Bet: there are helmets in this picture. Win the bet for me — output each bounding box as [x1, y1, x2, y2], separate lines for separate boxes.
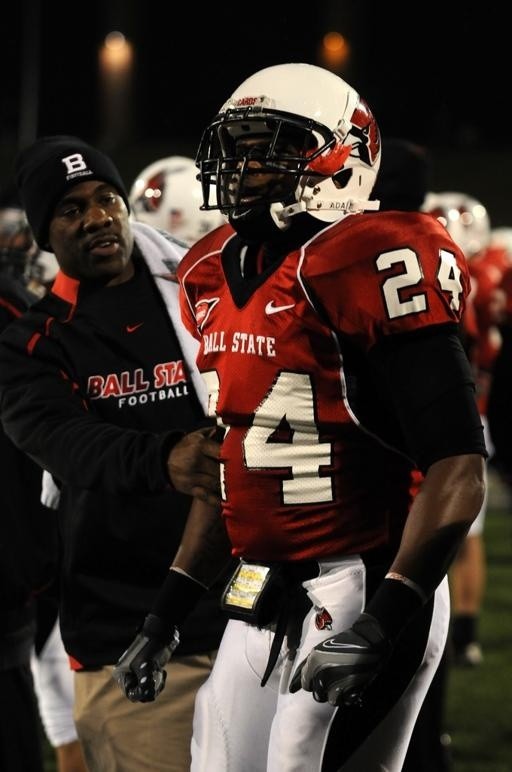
[418, 189, 492, 260]
[128, 156, 232, 252]
[195, 63, 385, 233]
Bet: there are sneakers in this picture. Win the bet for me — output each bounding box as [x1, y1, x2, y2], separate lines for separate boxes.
[453, 639, 485, 664]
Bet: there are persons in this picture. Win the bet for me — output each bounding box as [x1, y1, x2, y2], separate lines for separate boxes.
[117, 64, 486, 772]
[0, 139, 235, 771]
[370, 139, 510, 772]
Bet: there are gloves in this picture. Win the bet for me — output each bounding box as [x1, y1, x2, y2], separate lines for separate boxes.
[288, 611, 395, 712]
[113, 610, 180, 703]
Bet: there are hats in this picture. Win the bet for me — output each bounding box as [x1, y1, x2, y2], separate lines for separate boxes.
[23, 138, 130, 252]
